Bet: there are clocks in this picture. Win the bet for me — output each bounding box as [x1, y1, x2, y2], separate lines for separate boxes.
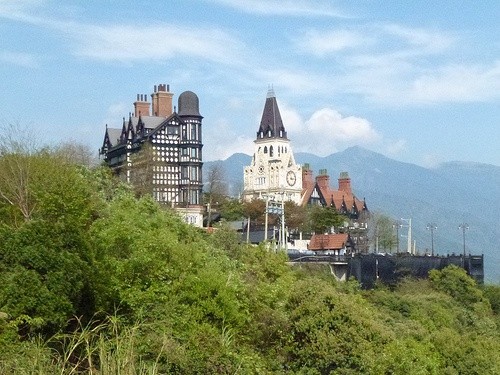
[287, 171, 296, 187]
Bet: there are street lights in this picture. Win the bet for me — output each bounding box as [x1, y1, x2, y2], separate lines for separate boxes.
[352, 218, 468, 255]
